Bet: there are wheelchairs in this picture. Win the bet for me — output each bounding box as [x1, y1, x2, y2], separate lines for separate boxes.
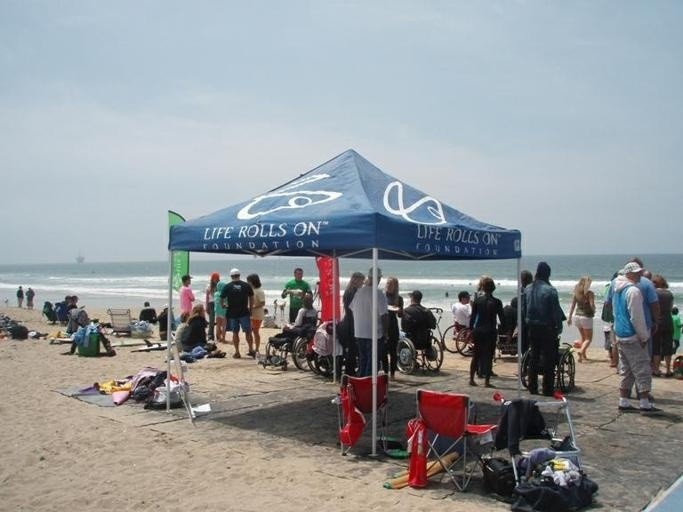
[256, 310, 360, 378]
[394, 306, 443, 372]
[442, 321, 477, 357]
[520, 340, 578, 394]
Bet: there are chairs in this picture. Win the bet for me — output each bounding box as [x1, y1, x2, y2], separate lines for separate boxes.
[406, 388, 499, 492]
[107, 308, 138, 337]
[42, 302, 99, 323]
[330, 371, 389, 457]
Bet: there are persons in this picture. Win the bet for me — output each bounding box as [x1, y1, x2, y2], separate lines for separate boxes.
[568, 275, 596, 362]
[175, 276, 207, 351]
[140, 301, 174, 340]
[336, 268, 403, 380]
[401, 290, 436, 349]
[62, 295, 71, 306]
[25, 288, 36, 310]
[206, 268, 265, 358]
[281, 268, 317, 339]
[69, 296, 79, 310]
[16, 286, 23, 308]
[601, 260, 682, 415]
[452, 261, 567, 396]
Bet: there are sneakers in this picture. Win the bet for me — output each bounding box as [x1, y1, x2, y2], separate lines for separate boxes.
[618, 405, 640, 413]
[639, 406, 663, 415]
[247, 352, 255, 356]
[666, 373, 676, 378]
[232, 352, 241, 358]
[469, 371, 498, 388]
[653, 371, 663, 377]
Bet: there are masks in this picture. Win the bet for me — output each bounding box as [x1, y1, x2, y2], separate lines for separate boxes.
[409, 291, 422, 298]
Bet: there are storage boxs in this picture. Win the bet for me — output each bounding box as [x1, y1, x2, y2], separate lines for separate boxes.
[77, 332, 101, 356]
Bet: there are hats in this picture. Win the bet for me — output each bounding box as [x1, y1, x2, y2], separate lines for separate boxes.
[625, 262, 644, 274]
[458, 291, 472, 297]
[230, 268, 240, 275]
